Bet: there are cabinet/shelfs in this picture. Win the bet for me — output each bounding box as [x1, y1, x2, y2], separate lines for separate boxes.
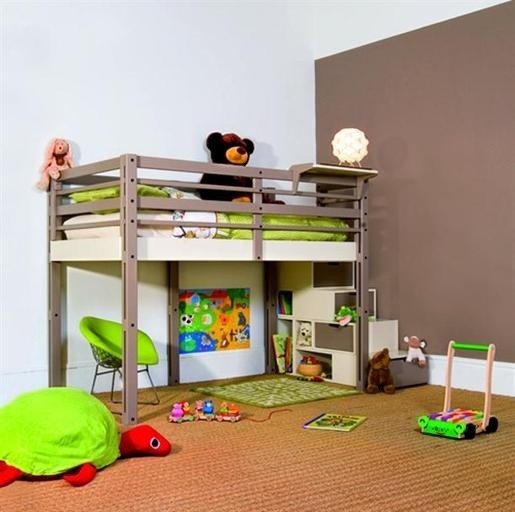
[272, 261, 430, 392]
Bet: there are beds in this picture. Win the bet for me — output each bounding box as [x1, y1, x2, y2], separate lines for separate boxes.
[47, 153, 370, 426]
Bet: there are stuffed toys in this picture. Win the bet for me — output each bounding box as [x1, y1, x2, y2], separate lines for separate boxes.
[198, 131, 287, 215]
[366, 347, 396, 395]
[403, 334, 427, 370]
[0, 386, 172, 488]
[35, 136, 77, 192]
[297, 323, 313, 347]
[334, 304, 375, 328]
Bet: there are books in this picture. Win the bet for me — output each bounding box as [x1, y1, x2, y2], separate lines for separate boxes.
[302, 412, 368, 433]
[271, 291, 294, 376]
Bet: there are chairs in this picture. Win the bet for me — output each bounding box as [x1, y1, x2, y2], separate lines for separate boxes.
[79, 315, 159, 416]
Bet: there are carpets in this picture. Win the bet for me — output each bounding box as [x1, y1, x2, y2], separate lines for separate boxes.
[188, 377, 360, 407]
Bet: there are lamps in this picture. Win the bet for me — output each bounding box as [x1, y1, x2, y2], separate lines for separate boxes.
[330, 128, 371, 167]
